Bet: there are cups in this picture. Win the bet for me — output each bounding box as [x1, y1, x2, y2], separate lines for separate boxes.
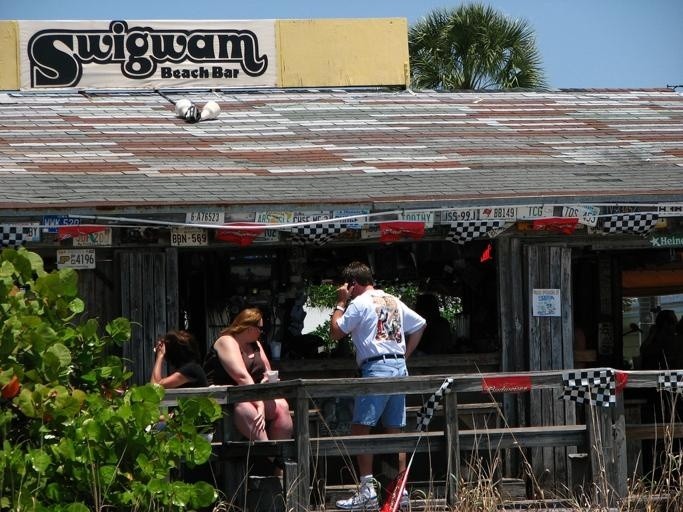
[268, 371, 278, 384]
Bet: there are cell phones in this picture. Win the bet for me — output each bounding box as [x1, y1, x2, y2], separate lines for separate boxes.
[346, 279, 355, 291]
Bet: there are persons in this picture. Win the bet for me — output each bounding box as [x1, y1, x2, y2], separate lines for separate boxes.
[641, 306, 683, 421]
[212, 306, 296, 475]
[329, 260, 428, 510]
[144, 327, 216, 482]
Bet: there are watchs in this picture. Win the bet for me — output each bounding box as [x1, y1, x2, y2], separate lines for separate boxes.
[333, 304, 346, 311]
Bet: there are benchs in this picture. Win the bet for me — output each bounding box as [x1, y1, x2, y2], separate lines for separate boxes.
[133, 368, 682, 508]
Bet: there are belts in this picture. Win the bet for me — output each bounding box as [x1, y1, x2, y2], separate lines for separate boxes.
[363, 355, 404, 364]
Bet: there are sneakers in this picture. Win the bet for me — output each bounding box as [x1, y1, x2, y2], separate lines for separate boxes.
[336, 492, 379, 509]
[399, 489, 409, 506]
